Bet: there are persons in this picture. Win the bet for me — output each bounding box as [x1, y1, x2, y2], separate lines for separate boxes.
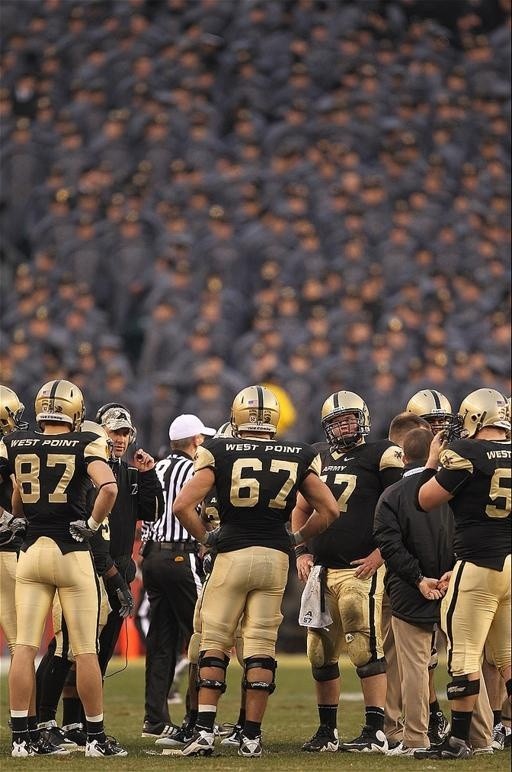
[382, 414, 432, 749]
[374, 430, 495, 759]
[38, 419, 136, 751]
[1, 2, 512, 389]
[172, 385, 341, 757]
[406, 391, 453, 747]
[0, 380, 128, 758]
[140, 412, 218, 738]
[290, 389, 403, 752]
[64, 405, 166, 748]
[416, 390, 512, 758]
[2, 386, 68, 755]
[155, 419, 281, 757]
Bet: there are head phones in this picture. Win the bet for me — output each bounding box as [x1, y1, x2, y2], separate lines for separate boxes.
[95, 403, 137, 442]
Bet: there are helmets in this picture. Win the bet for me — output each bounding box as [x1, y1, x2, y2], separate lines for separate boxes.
[231, 386, 280, 433]
[321, 391, 371, 448]
[449, 387, 510, 437]
[406, 389, 453, 439]
[212, 422, 234, 440]
[35, 380, 85, 431]
[0, 385, 29, 435]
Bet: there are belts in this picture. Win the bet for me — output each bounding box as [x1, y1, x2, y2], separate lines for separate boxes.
[160, 541, 193, 553]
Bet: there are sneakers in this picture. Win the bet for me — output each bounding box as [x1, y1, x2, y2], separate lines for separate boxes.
[212, 719, 234, 737]
[470, 746, 494, 756]
[37, 720, 78, 750]
[85, 736, 128, 758]
[154, 723, 198, 746]
[340, 725, 389, 753]
[220, 725, 243, 747]
[430, 709, 451, 745]
[382, 742, 429, 757]
[181, 730, 215, 756]
[141, 719, 181, 739]
[414, 737, 473, 761]
[301, 725, 340, 754]
[237, 735, 263, 758]
[9, 730, 52, 759]
[28, 728, 70, 757]
[490, 723, 511, 751]
[60, 725, 88, 752]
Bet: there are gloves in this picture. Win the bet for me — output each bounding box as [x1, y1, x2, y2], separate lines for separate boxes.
[1, 510, 28, 536]
[110, 572, 136, 620]
[201, 529, 221, 549]
[67, 520, 94, 543]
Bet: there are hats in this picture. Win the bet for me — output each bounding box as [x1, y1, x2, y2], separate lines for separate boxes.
[168, 414, 216, 440]
[96, 403, 134, 432]
